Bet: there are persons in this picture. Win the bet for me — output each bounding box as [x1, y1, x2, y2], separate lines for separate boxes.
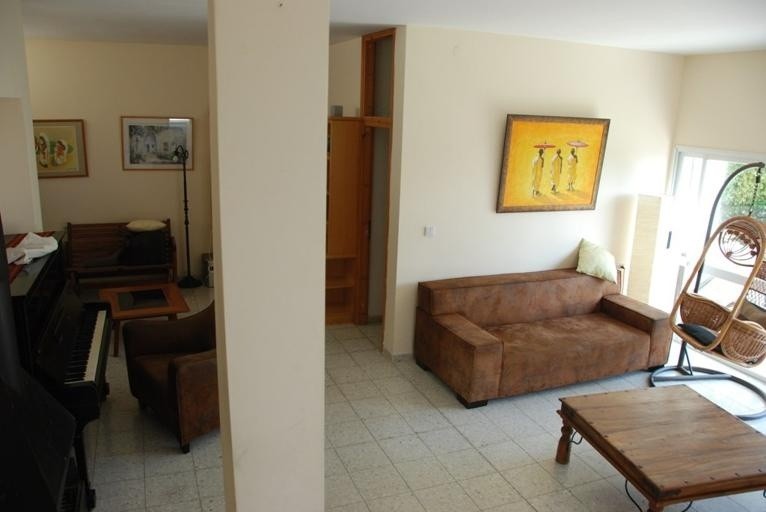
[566, 148, 578, 191]
[531, 148, 544, 196]
[550, 148, 563, 192]
[56, 140, 65, 160]
[38, 136, 48, 168]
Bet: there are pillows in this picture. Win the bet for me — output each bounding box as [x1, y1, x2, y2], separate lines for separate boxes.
[576, 237, 618, 283]
[677, 323, 721, 352]
[126, 220, 167, 232]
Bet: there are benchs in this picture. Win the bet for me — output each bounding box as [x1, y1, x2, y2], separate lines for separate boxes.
[67, 219, 178, 298]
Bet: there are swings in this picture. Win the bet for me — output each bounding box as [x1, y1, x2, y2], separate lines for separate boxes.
[669, 165, 765, 369]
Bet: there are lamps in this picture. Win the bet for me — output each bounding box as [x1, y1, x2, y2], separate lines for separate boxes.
[172, 144, 203, 289]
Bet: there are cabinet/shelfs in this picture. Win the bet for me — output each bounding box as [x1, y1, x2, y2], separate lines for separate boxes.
[325, 116, 359, 326]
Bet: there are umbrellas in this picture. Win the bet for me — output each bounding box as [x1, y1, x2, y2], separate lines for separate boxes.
[533, 143, 555, 148]
[566, 140, 589, 147]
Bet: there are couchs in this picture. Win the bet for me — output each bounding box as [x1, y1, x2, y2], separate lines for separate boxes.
[413, 267, 677, 410]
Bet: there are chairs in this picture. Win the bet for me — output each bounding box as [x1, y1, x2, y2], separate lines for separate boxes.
[122, 300, 220, 454]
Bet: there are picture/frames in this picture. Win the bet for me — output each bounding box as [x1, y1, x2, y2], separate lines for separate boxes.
[495, 114, 611, 214]
[32, 118, 89, 179]
[120, 116, 194, 171]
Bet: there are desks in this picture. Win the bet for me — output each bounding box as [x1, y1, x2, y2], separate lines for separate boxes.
[98, 282, 190, 357]
